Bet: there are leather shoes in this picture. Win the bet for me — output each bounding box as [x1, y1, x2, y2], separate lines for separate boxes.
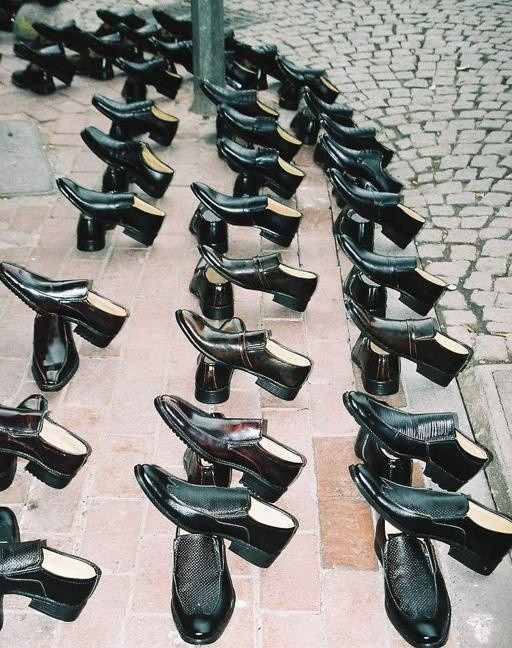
[0, 234, 512, 648]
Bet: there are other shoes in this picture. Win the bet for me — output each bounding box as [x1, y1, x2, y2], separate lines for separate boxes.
[9, 7, 425, 253]
